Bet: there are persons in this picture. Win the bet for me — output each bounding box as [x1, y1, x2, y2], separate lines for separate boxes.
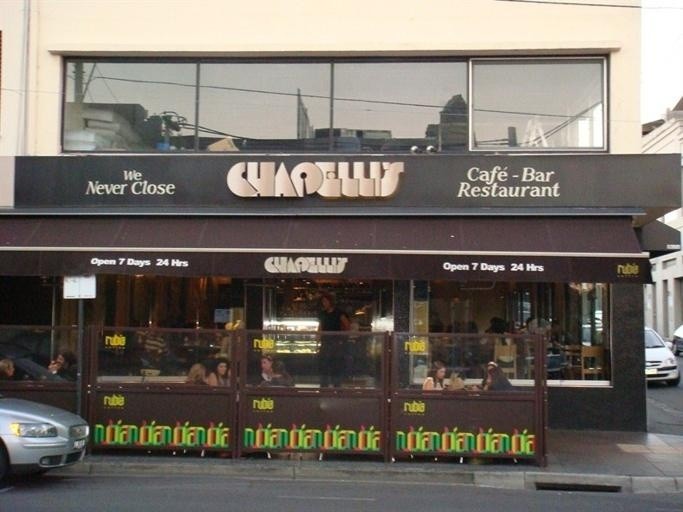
[0, 357, 17, 380]
[254, 352, 294, 386]
[184, 362, 209, 385]
[420, 359, 448, 391]
[43, 350, 78, 382]
[429, 311, 564, 377]
[469, 367, 512, 392]
[311, 293, 352, 388]
[204, 356, 232, 387]
[260, 360, 292, 386]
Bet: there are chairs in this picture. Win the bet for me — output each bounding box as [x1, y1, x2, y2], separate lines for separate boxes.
[494, 344, 607, 380]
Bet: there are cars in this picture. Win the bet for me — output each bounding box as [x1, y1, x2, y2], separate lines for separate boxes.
[0, 396, 90, 486]
[517, 301, 531, 321]
[583, 310, 605, 344]
[645, 325, 683, 387]
